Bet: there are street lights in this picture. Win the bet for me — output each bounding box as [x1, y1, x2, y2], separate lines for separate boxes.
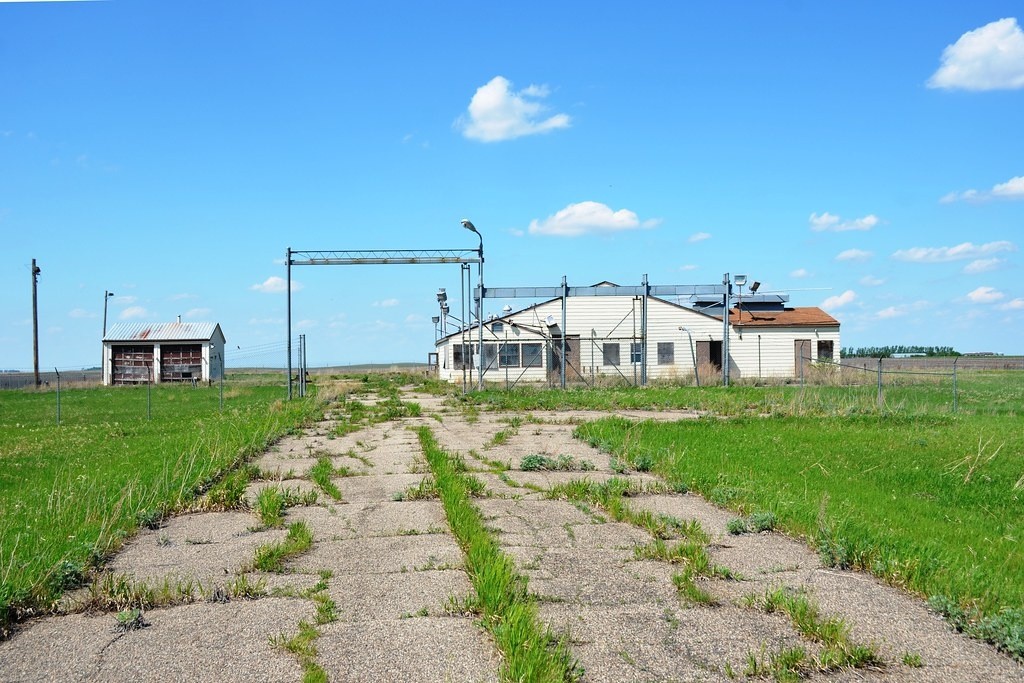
[101, 290, 114, 381]
[432, 316, 440, 342]
[460, 219, 483, 388]
